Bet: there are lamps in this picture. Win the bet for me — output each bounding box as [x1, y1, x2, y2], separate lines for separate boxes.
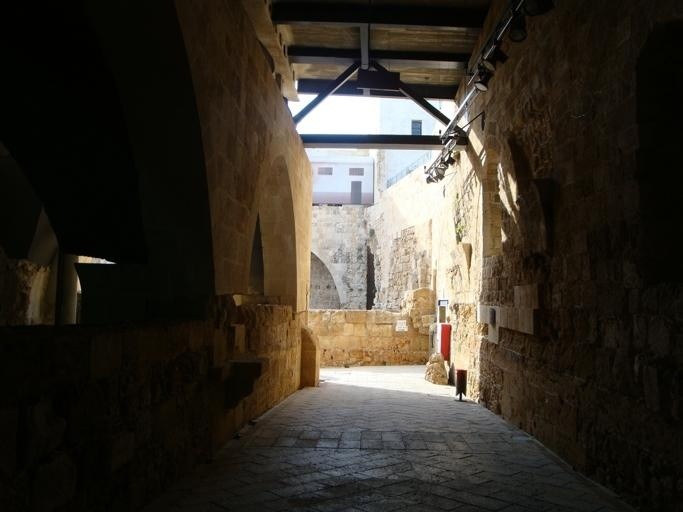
[424, 0, 554, 185]
